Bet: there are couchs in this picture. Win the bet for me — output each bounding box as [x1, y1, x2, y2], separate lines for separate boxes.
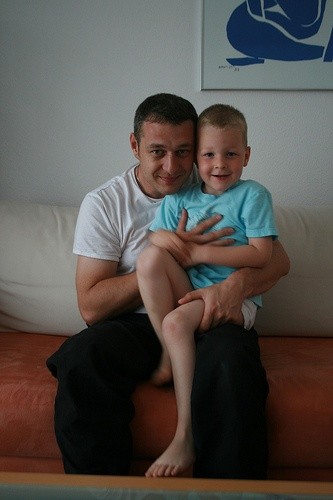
[0, 202, 333, 480]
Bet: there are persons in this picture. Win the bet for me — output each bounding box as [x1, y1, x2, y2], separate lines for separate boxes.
[135, 105, 278, 478]
[46, 92, 289, 480]
